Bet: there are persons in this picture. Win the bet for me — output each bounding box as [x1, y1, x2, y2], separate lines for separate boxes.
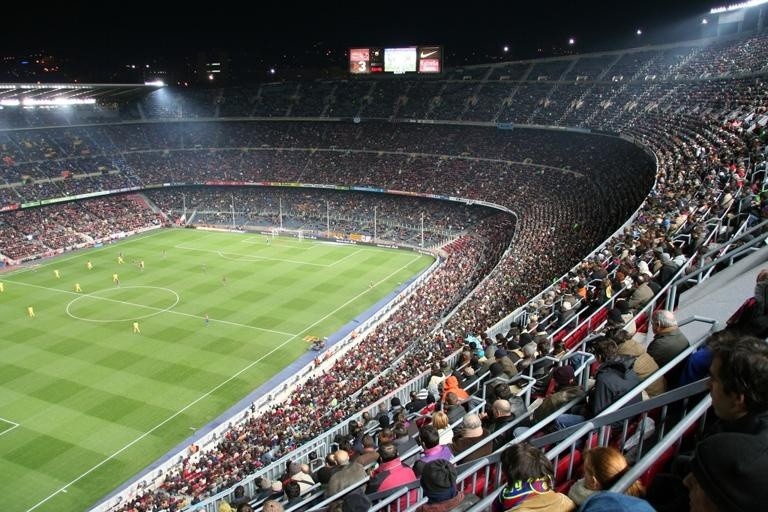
[27, 306, 35, 317]
[75, 283, 82, 293]
[108, 275, 768, 510]
[0, 283, 4, 293]
[0, 34, 768, 273]
[54, 269, 61, 279]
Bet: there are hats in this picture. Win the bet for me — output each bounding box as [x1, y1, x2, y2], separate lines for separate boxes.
[580, 493, 656, 512]
[554, 366, 576, 386]
[420, 458, 459, 498]
[289, 463, 302, 474]
[689, 432, 767, 511]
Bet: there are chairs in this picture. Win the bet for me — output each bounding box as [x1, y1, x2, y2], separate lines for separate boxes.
[218, 159, 768, 512]
